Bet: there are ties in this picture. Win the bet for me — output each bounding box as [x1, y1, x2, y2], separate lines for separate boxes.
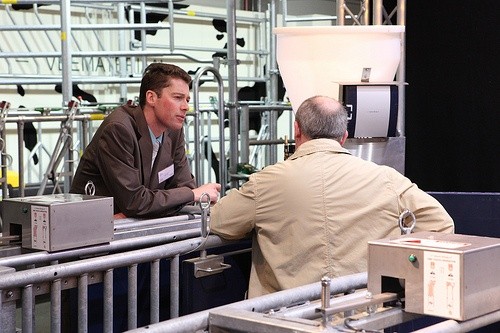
[152, 141, 159, 173]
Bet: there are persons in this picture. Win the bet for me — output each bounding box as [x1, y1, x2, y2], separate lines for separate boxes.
[208, 95, 456, 333]
[68, 62, 222, 220]
